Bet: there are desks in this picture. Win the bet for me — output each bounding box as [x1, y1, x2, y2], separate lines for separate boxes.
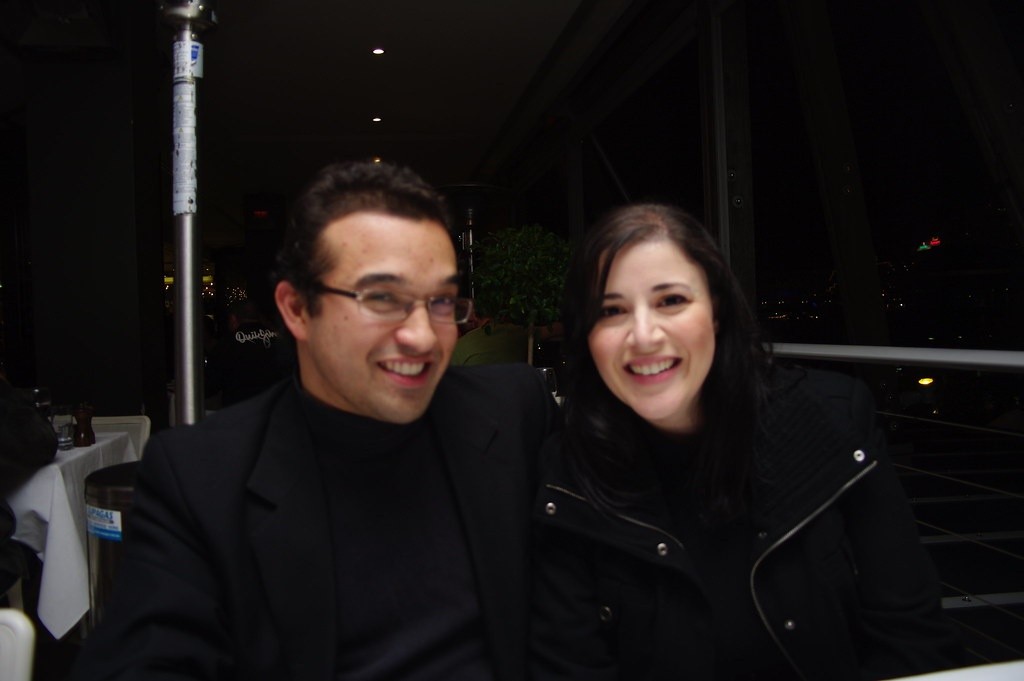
[4, 430, 137, 643]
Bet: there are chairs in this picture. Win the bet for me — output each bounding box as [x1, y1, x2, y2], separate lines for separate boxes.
[48, 416, 151, 461]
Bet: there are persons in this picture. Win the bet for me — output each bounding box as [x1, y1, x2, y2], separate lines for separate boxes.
[0, 368, 81, 681]
[450, 274, 543, 367]
[74, 157, 564, 681]
[520, 203, 969, 681]
[206, 297, 286, 398]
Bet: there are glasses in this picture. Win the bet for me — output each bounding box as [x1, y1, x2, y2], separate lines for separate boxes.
[315, 284, 473, 323]
